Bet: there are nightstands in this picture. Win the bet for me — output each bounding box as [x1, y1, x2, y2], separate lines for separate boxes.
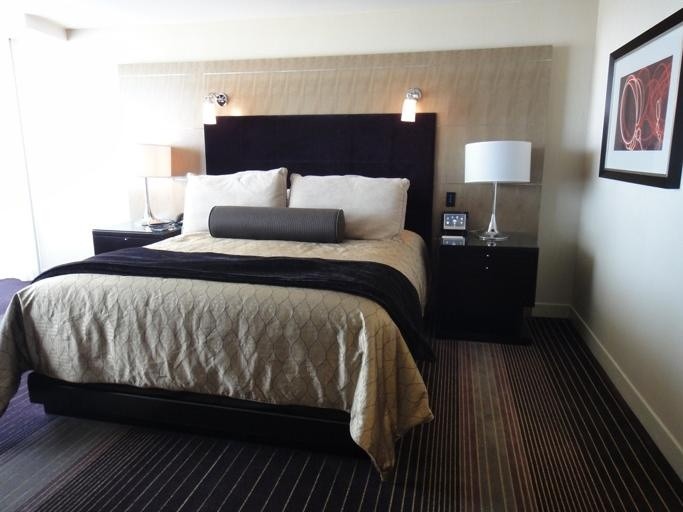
[91, 227, 183, 255]
[439, 235, 540, 344]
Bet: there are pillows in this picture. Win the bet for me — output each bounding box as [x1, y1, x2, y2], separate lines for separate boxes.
[183, 169, 409, 245]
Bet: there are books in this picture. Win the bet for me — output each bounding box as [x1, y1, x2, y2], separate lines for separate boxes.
[148, 220, 173, 232]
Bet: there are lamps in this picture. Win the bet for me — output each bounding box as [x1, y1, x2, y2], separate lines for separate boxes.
[201, 90, 423, 123]
[115, 143, 172, 232]
[464, 142, 532, 241]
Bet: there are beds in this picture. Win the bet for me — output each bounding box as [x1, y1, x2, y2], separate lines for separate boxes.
[15, 112, 437, 449]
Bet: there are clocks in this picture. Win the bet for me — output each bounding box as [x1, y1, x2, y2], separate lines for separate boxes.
[441, 210, 467, 238]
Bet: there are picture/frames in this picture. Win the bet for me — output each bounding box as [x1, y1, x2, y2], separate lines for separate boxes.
[598, 7, 682, 190]
